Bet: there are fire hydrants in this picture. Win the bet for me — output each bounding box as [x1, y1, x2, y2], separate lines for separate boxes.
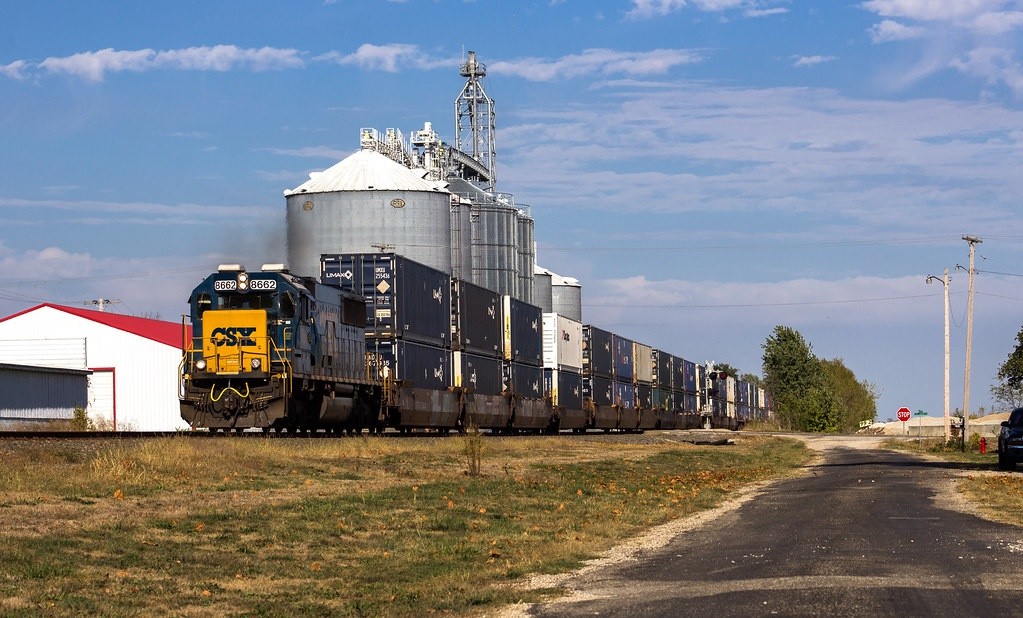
[979, 437, 987, 453]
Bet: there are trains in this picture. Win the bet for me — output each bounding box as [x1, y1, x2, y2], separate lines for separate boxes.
[178, 252, 773, 436]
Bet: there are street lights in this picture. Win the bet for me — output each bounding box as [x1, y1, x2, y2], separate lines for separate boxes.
[955, 264, 974, 452]
[926, 274, 950, 447]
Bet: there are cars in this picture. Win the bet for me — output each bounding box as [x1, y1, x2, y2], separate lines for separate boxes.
[998, 407, 1023, 471]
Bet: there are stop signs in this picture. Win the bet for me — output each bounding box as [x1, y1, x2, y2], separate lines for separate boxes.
[896, 407, 911, 422]
